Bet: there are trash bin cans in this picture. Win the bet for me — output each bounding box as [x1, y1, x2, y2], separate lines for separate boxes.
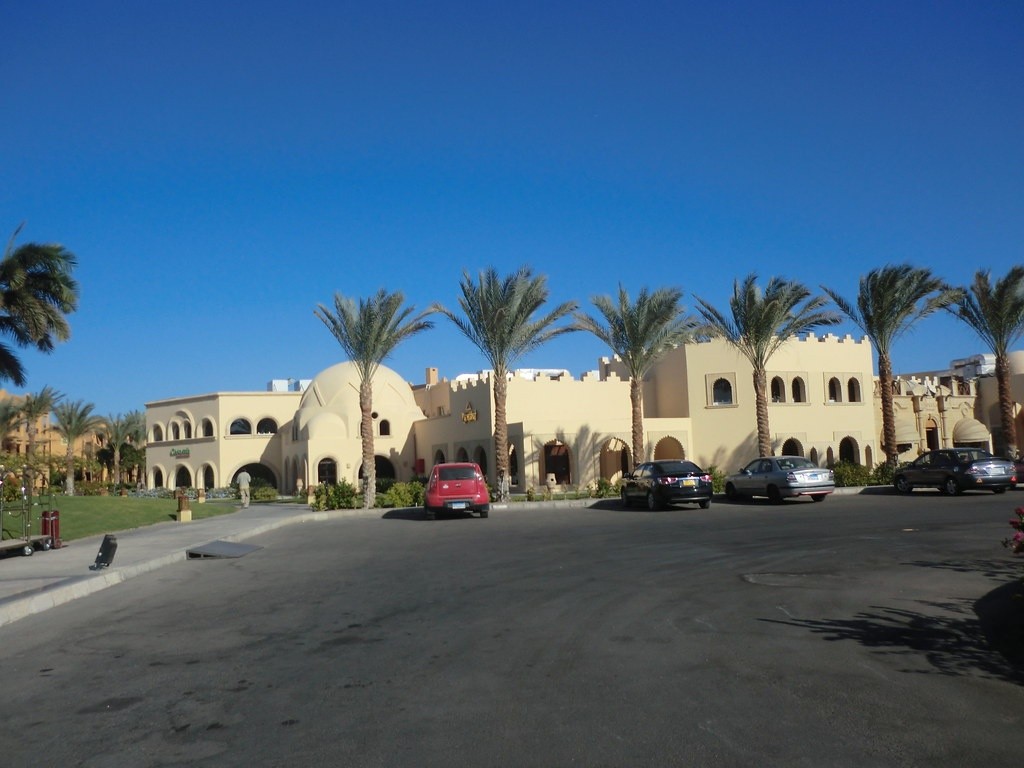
[41, 511, 59, 549]
[1014, 460, 1024, 483]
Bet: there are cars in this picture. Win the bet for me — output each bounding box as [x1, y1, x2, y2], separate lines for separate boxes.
[893, 447, 1017, 496]
[722, 456, 835, 505]
[620, 459, 714, 511]
[423, 460, 491, 520]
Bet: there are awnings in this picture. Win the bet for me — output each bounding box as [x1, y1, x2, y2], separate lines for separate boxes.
[953, 418, 990, 443]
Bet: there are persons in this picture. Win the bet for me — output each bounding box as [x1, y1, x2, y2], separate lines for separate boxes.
[236, 467, 252, 508]
